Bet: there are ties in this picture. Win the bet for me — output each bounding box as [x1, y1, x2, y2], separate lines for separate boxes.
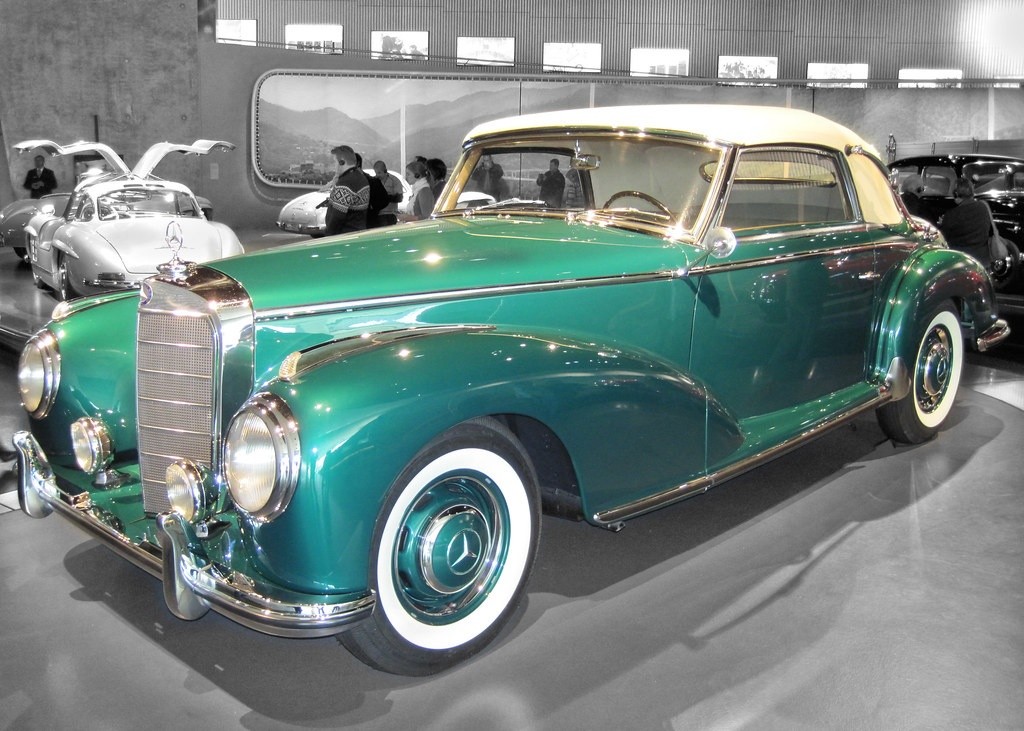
[38, 168, 41, 177]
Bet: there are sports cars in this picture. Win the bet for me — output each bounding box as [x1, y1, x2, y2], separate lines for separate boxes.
[1, 136, 248, 299]
[275, 168, 410, 241]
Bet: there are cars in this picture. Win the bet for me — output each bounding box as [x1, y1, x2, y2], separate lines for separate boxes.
[882, 152, 1024, 322]
[0, 104, 1011, 682]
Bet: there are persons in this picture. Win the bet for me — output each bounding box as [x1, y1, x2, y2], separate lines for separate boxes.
[373, 161, 403, 226]
[23, 155, 57, 199]
[356, 153, 389, 229]
[380, 34, 425, 60]
[724, 60, 765, 79]
[899, 174, 925, 217]
[399, 162, 435, 222]
[936, 177, 992, 274]
[425, 158, 447, 201]
[415, 156, 427, 164]
[472, 154, 503, 197]
[536, 158, 565, 207]
[325, 145, 370, 235]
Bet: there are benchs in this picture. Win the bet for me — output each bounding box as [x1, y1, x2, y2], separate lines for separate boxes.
[680, 203, 847, 232]
